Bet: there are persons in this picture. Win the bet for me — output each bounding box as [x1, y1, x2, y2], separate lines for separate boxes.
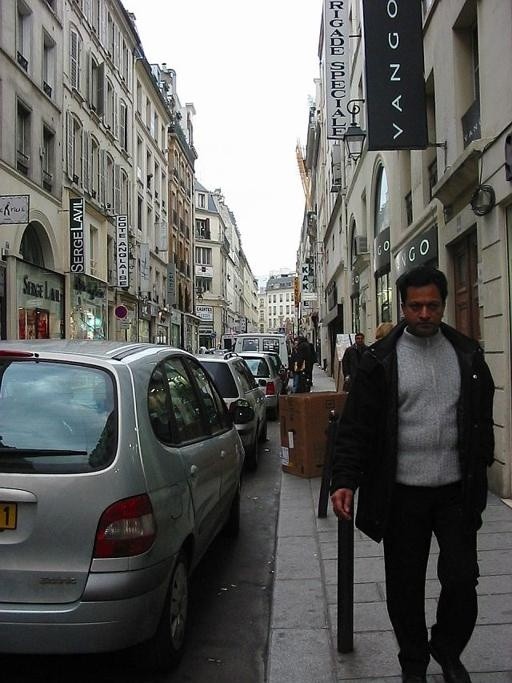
[291, 336, 316, 392]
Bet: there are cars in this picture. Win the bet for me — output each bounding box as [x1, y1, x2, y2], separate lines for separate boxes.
[190, 349, 290, 448]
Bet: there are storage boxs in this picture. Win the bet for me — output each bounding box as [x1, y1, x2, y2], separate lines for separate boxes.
[331, 331, 358, 392]
[277, 390, 350, 479]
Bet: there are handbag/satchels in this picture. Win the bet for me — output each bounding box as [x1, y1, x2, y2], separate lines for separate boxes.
[343, 377, 352, 392]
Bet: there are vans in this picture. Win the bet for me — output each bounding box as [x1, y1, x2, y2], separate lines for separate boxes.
[233, 332, 292, 375]
[0, 337, 246, 671]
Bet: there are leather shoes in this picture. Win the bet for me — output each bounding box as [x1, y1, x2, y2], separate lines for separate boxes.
[399, 640, 471, 683]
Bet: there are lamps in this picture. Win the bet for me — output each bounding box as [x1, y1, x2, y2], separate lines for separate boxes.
[341, 98, 367, 165]
